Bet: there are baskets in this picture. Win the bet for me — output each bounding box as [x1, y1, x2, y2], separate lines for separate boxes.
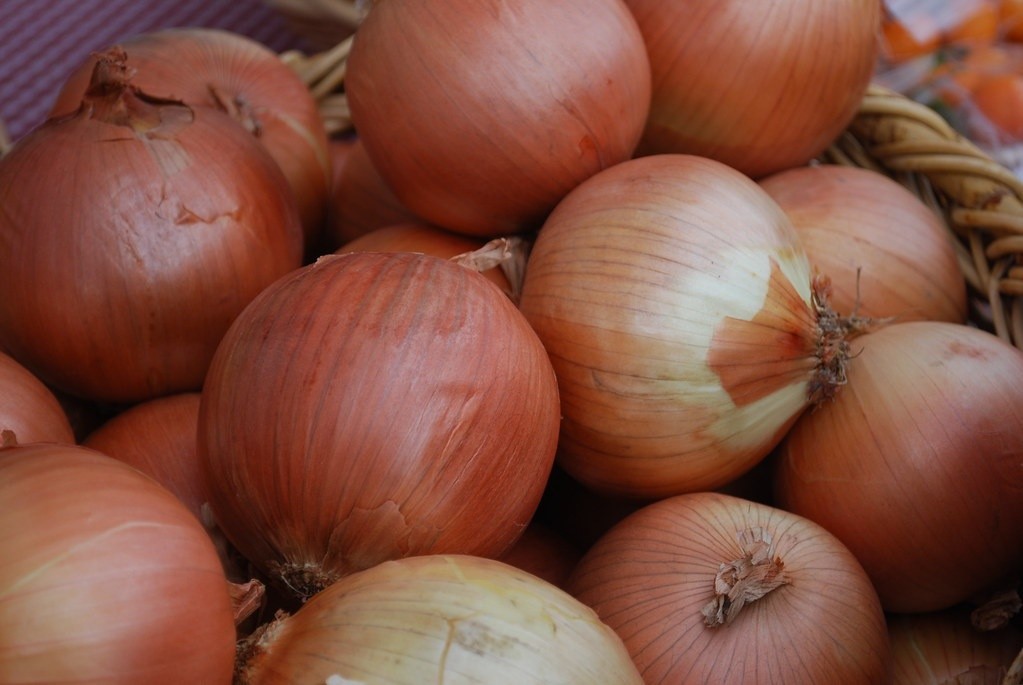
[279, 39, 1022, 685]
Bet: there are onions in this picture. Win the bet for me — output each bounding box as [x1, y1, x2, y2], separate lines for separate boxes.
[0, 0, 1023, 685]
